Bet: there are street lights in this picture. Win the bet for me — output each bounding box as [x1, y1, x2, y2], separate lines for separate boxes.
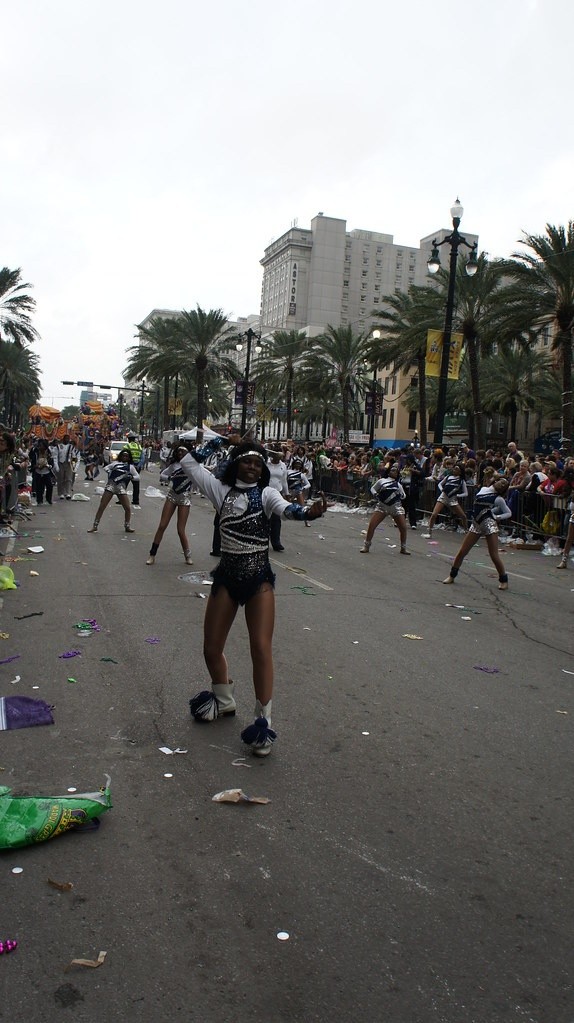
[204, 383, 213, 420]
[426, 196, 479, 444]
[368, 329, 381, 448]
[115, 393, 126, 418]
[235, 327, 263, 441]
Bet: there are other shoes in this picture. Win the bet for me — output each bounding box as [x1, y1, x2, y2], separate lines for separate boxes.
[394, 523, 397, 527]
[273, 544, 284, 551]
[87, 527, 97, 532]
[411, 525, 417, 530]
[145, 554, 156, 564]
[184, 551, 193, 565]
[66, 494, 71, 500]
[131, 501, 139, 505]
[37, 501, 43, 506]
[116, 501, 122, 504]
[124, 526, 136, 532]
[46, 499, 53, 505]
[60, 494, 64, 499]
[306, 523, 312, 527]
[210, 549, 222, 557]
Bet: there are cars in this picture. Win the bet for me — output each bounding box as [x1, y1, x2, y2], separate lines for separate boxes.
[101, 440, 130, 467]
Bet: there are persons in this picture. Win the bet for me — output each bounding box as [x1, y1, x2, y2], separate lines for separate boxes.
[84, 449, 99, 481]
[116, 436, 145, 505]
[556, 494, 574, 568]
[146, 444, 195, 565]
[0, 428, 81, 527]
[87, 450, 141, 533]
[35, 415, 64, 425]
[443, 476, 512, 590]
[139, 439, 236, 498]
[262, 438, 574, 551]
[360, 466, 411, 555]
[80, 403, 90, 415]
[178, 441, 336, 755]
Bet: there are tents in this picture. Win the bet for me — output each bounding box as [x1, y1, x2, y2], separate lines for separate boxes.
[178, 422, 221, 440]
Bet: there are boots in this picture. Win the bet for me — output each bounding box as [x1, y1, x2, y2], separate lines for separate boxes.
[188, 680, 236, 722]
[360, 541, 371, 553]
[427, 527, 433, 539]
[400, 544, 411, 555]
[556, 554, 569, 568]
[240, 699, 277, 756]
[442, 567, 459, 583]
[498, 573, 508, 589]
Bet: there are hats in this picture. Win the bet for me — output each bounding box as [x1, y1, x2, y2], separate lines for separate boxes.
[404, 454, 415, 460]
[544, 460, 557, 468]
[126, 430, 138, 437]
[459, 443, 468, 449]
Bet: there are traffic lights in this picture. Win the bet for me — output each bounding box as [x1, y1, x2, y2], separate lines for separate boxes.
[293, 408, 302, 414]
[271, 408, 280, 412]
